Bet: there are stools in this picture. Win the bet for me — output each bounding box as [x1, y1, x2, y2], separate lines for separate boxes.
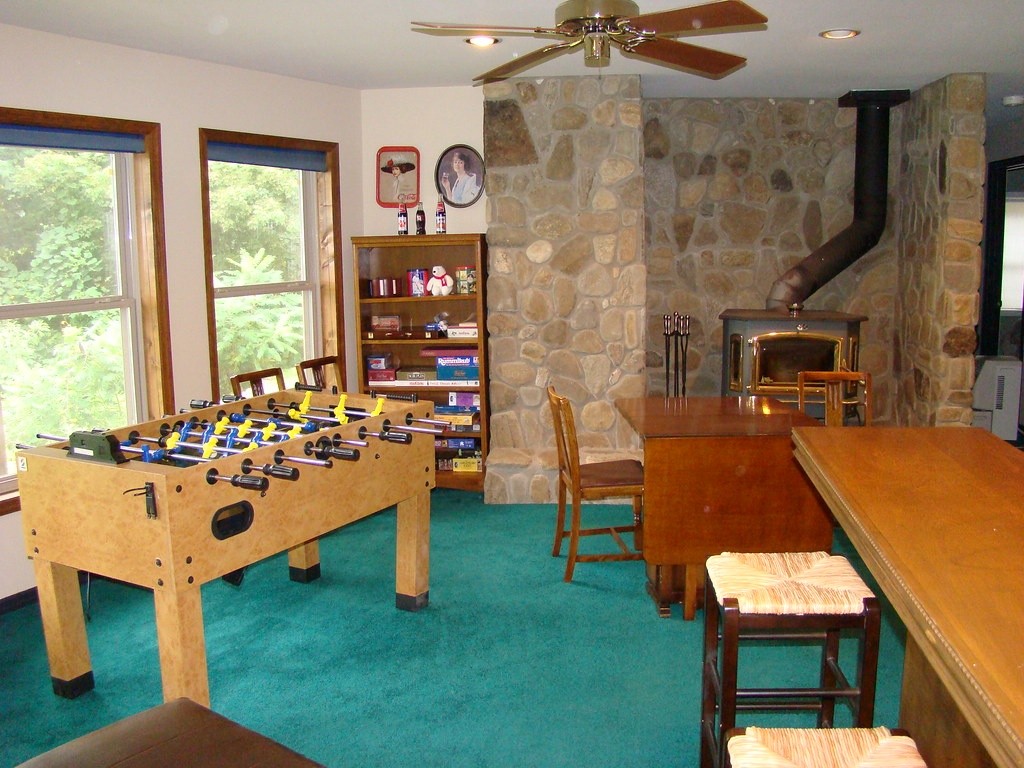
[701, 550, 879, 768]
[720, 726, 931, 768]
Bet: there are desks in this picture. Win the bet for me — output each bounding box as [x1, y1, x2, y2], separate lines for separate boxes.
[12, 696, 330, 768]
[612, 396, 834, 622]
[790, 425, 1024, 768]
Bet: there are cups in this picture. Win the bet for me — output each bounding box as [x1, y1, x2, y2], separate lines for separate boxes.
[443, 172, 449, 181]
[404, 268, 430, 297]
[454, 265, 476, 294]
[370, 277, 396, 297]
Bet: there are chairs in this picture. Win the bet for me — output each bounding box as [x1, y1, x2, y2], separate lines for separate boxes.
[798, 371, 872, 429]
[229, 367, 286, 401]
[546, 384, 643, 581]
[296, 356, 344, 392]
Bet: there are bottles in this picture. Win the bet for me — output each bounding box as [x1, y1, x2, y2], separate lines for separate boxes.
[415, 201, 426, 235]
[436, 194, 446, 234]
[397, 194, 409, 235]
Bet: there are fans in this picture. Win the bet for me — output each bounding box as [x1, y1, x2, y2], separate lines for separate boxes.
[410, 0, 768, 79]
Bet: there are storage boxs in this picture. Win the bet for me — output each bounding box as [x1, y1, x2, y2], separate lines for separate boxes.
[366, 346, 480, 380]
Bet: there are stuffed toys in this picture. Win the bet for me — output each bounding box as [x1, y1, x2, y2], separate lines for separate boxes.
[427, 265, 453, 296]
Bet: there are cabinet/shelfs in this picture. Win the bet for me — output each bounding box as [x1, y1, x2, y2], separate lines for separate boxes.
[350, 233, 486, 492]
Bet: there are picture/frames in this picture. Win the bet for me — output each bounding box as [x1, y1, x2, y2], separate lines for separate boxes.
[376, 145, 421, 208]
[434, 144, 486, 208]
